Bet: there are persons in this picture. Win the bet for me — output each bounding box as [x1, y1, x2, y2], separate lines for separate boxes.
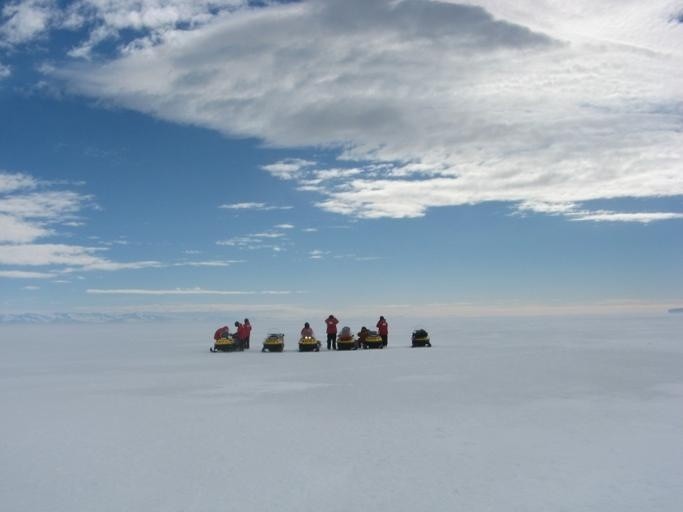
[300, 321, 314, 337]
[243, 317, 252, 350]
[209, 325, 230, 352]
[232, 320, 247, 352]
[337, 325, 355, 342]
[324, 314, 339, 350]
[357, 326, 369, 350]
[375, 315, 389, 349]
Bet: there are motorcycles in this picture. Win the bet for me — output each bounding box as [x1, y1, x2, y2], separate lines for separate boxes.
[411, 329, 431, 347]
[298, 336, 319, 352]
[362, 331, 382, 349]
[261, 334, 284, 352]
[336, 334, 356, 351]
[209, 334, 240, 352]
